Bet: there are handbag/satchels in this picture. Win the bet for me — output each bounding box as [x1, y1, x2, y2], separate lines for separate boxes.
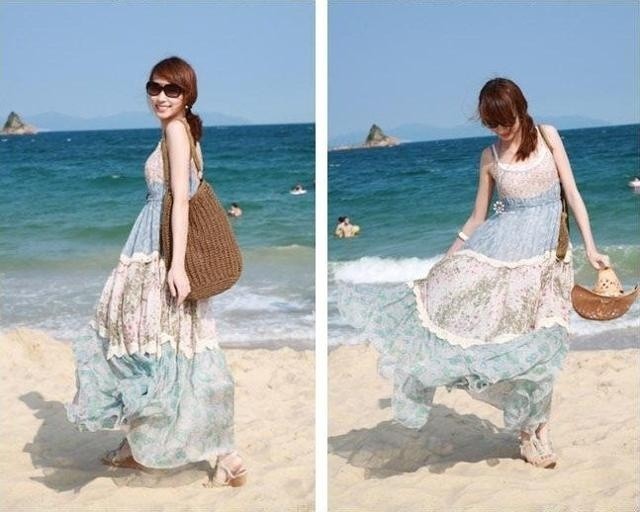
[161, 178, 243, 303]
[556, 212, 569, 263]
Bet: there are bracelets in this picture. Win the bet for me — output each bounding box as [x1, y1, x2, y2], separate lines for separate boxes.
[458, 232, 469, 241]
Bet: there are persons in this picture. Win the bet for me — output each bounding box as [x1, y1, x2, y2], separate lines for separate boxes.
[226, 201, 242, 218]
[628, 176, 639, 193]
[336, 77, 610, 470]
[64, 56, 249, 488]
[292, 185, 303, 196]
[336, 214, 354, 238]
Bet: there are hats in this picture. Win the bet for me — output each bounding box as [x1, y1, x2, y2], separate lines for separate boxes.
[570, 268, 640, 321]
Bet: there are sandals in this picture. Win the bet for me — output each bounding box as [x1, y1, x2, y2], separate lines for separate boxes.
[101, 438, 144, 470]
[203, 451, 247, 488]
[518, 429, 556, 470]
